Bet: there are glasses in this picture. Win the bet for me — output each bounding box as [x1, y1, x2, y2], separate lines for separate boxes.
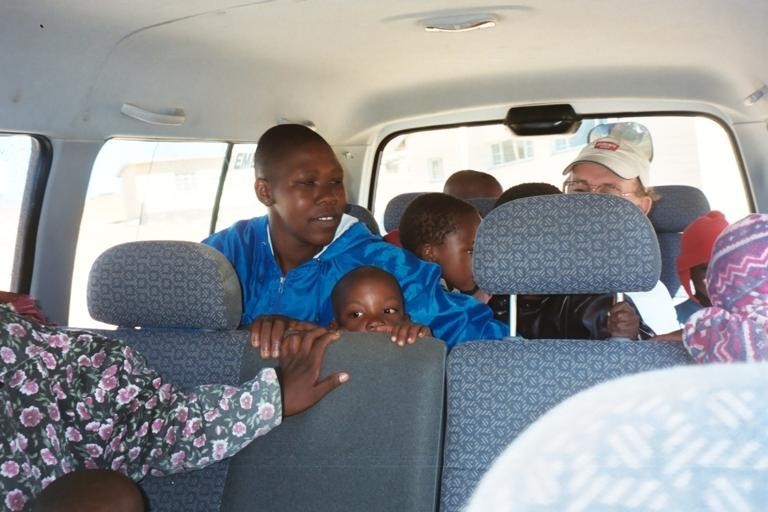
[562, 179, 633, 201]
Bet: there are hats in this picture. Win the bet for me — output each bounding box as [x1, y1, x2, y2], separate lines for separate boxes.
[466, 361, 768, 511]
[675, 210, 731, 305]
[562, 135, 652, 194]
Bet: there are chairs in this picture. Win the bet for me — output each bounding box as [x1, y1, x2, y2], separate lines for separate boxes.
[384, 191, 499, 236]
[79, 241, 448, 512]
[648, 186, 712, 322]
[438, 193, 698, 512]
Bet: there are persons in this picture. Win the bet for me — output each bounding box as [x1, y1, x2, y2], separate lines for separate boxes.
[0, 286, 348, 512]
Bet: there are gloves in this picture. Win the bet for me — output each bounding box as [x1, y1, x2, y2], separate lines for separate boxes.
[681, 211, 767, 362]
